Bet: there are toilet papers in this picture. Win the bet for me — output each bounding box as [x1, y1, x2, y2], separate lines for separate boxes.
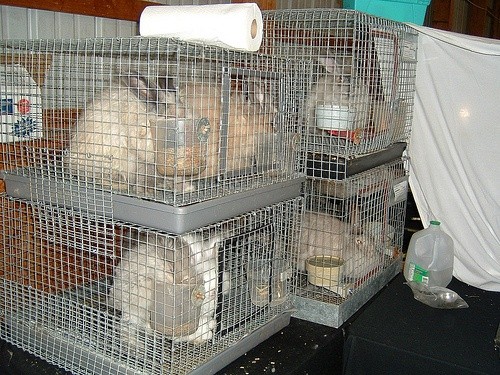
[139, 3, 264, 52]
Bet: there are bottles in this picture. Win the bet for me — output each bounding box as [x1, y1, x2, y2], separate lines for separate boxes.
[402, 221, 455, 291]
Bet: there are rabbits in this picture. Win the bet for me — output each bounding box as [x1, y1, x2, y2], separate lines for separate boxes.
[178, 80, 280, 180]
[66, 69, 195, 198]
[303, 49, 372, 134]
[108, 229, 235, 360]
[296, 205, 382, 278]
